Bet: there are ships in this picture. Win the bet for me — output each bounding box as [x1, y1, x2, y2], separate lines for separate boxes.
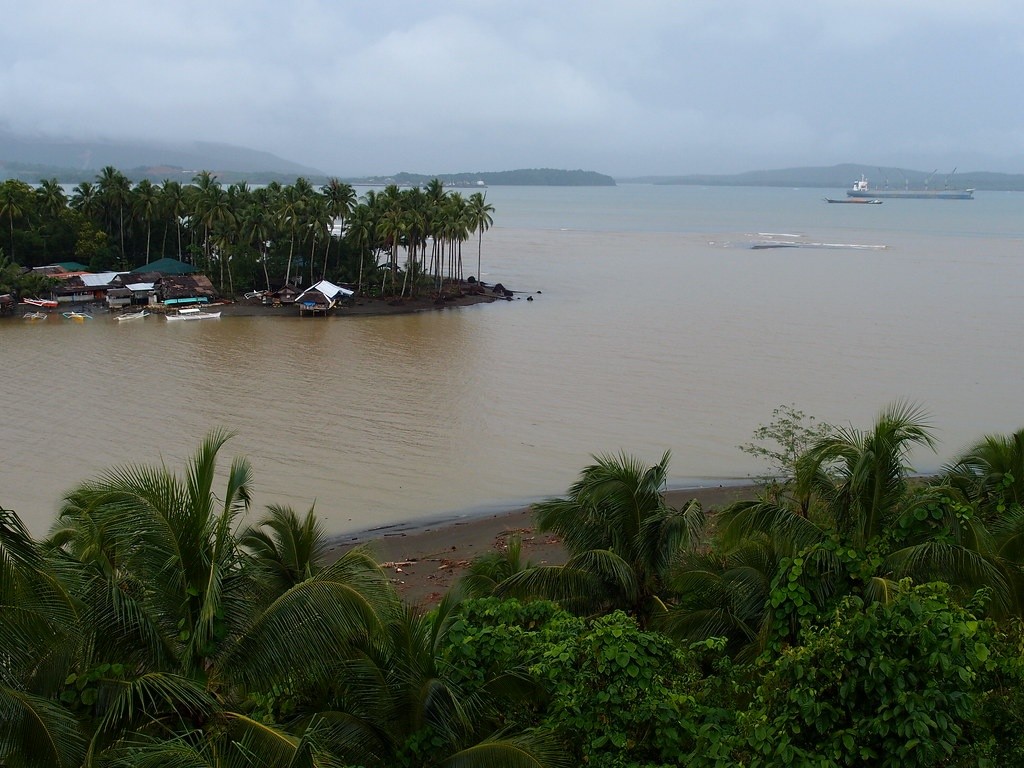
[847, 168, 975, 199]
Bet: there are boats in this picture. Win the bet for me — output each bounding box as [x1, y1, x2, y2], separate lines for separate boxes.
[826, 197, 882, 203]
[22, 312, 46, 320]
[25, 296, 58, 307]
[757, 232, 887, 248]
[113, 310, 149, 321]
[165, 308, 221, 321]
[64, 310, 93, 321]
